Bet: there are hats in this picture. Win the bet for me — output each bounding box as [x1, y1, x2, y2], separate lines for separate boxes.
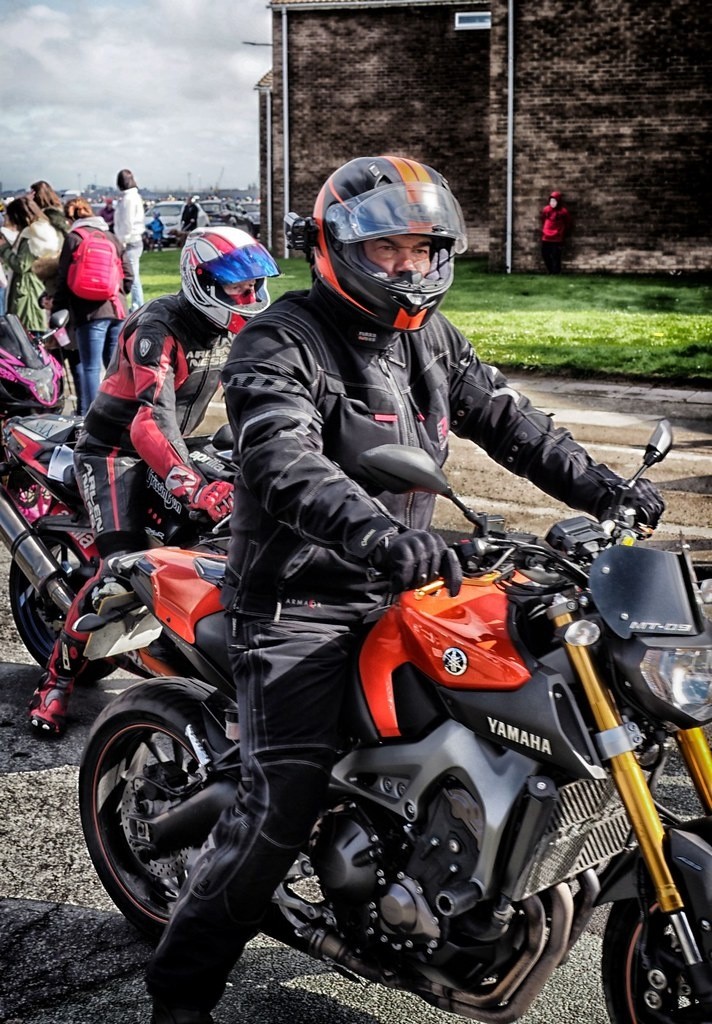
[106, 199, 112, 203]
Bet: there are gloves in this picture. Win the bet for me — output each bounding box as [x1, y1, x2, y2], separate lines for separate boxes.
[367, 529, 462, 598]
[598, 478, 666, 540]
[165, 464, 235, 523]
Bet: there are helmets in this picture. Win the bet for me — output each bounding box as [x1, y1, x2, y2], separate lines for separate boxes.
[153, 211, 160, 217]
[180, 226, 271, 335]
[309, 157, 456, 332]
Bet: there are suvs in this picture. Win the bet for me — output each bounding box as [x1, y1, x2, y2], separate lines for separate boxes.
[200, 196, 256, 241]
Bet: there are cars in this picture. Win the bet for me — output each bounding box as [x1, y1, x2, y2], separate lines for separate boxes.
[144, 199, 210, 248]
[239, 201, 261, 229]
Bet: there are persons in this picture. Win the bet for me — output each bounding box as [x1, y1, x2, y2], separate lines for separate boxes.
[0, 169, 283, 737]
[140, 154, 666, 1024]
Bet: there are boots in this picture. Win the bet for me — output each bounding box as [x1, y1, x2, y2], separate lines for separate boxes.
[28, 625, 90, 735]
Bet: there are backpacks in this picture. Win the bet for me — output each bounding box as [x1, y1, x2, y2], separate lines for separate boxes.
[66, 226, 123, 299]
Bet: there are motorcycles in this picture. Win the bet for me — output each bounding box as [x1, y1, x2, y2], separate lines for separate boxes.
[0, 307, 711, 1024]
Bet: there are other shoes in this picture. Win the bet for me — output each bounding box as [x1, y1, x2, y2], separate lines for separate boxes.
[150, 996, 214, 1024]
[71, 410, 77, 416]
